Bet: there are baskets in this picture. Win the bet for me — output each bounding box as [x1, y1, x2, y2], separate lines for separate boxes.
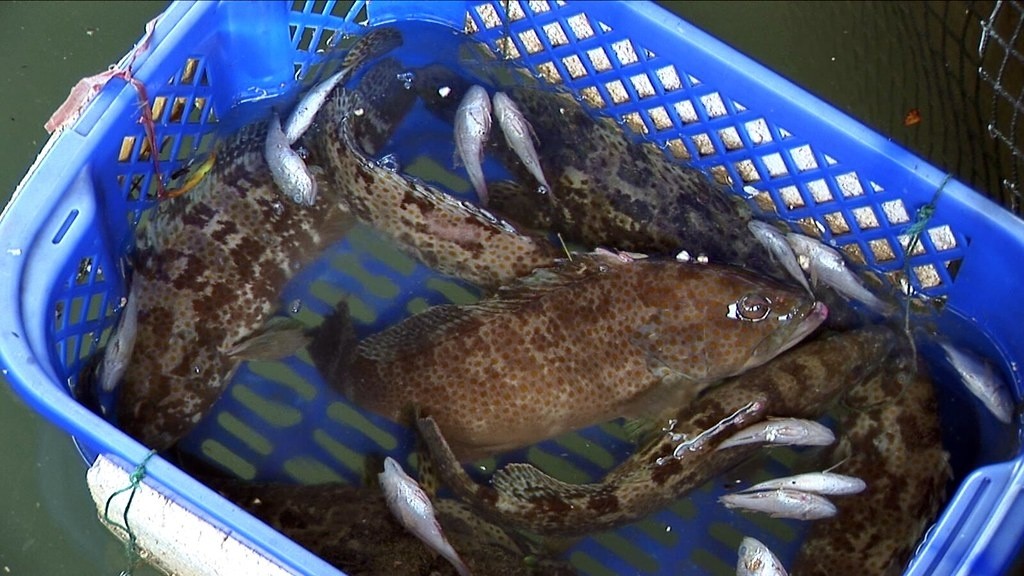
[0, 1, 1024, 576]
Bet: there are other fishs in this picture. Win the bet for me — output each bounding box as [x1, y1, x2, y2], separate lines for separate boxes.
[71, 22, 1015, 575]
